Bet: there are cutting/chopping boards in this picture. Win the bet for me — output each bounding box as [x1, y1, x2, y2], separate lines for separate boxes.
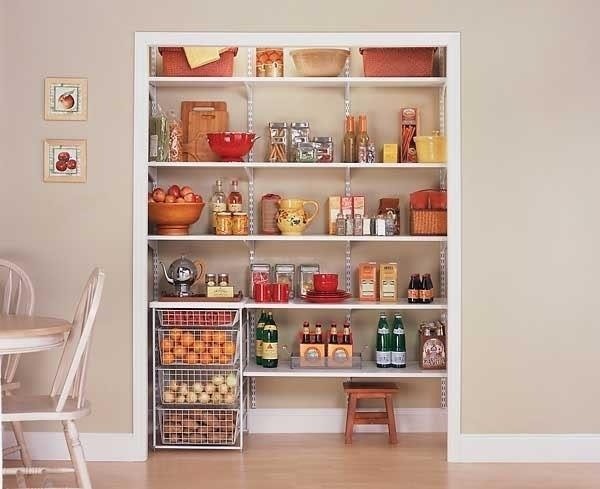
[176, 97, 228, 163]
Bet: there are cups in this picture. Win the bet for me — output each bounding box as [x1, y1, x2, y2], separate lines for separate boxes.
[412, 135, 447, 163]
[271, 284, 290, 303]
[251, 281, 271, 302]
[380, 208, 401, 234]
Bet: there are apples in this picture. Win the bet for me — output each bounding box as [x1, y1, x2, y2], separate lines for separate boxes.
[58, 94, 74, 109]
[56, 152, 76, 171]
[148, 184, 202, 203]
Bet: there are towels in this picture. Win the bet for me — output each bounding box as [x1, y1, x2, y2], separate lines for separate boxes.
[182, 46, 232, 70]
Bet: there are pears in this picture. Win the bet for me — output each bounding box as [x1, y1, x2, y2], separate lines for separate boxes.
[162, 375, 236, 404]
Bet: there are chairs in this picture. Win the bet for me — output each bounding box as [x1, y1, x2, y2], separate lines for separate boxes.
[0, 264, 108, 488]
[0, 257, 37, 469]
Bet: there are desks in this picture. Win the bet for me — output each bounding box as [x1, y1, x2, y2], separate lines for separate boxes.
[0, 310, 71, 488]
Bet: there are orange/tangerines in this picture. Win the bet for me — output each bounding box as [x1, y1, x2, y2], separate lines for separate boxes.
[161, 328, 235, 364]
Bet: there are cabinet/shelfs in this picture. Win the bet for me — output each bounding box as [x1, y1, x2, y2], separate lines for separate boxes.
[148, 46, 449, 432]
[151, 309, 250, 453]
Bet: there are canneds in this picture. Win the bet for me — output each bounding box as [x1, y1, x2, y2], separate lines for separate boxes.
[232, 212, 249, 235]
[216, 212, 232, 235]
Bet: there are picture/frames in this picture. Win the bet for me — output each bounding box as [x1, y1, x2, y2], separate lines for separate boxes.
[43, 75, 90, 122]
[42, 137, 88, 185]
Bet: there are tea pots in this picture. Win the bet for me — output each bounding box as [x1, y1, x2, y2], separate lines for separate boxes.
[156, 254, 204, 297]
[274, 198, 320, 235]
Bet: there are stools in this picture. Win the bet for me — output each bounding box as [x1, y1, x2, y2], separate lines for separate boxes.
[343, 380, 401, 446]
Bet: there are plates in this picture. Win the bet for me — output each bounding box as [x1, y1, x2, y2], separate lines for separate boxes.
[302, 291, 353, 305]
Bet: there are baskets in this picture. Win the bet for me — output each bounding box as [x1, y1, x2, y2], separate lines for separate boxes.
[157, 310, 241, 445]
[410, 189, 446, 236]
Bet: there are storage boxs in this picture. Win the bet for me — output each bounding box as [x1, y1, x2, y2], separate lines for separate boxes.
[419, 333, 447, 370]
[299, 332, 326, 369]
[328, 333, 354, 370]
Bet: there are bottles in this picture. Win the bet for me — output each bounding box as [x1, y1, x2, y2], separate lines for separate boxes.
[376, 311, 407, 369]
[255, 310, 278, 368]
[335, 213, 395, 236]
[301, 320, 312, 343]
[406, 273, 434, 304]
[343, 323, 350, 344]
[150, 116, 169, 162]
[250, 263, 319, 297]
[210, 179, 244, 235]
[268, 120, 334, 162]
[203, 273, 229, 286]
[314, 323, 323, 343]
[419, 319, 444, 337]
[343, 114, 369, 162]
[330, 323, 337, 343]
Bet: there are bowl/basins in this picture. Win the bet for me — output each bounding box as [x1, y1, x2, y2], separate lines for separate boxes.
[313, 273, 339, 292]
[203, 131, 256, 162]
[288, 49, 350, 77]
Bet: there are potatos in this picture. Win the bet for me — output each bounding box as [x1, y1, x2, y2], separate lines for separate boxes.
[164, 413, 237, 443]
